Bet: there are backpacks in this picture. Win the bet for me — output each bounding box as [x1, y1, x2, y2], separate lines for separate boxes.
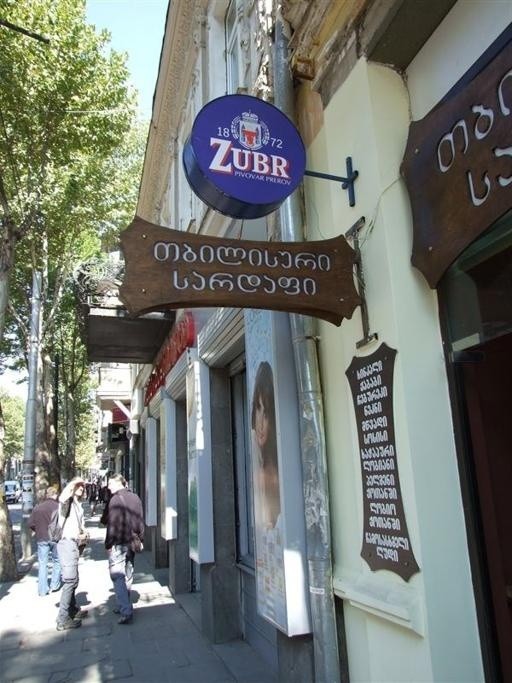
[47, 505, 70, 543]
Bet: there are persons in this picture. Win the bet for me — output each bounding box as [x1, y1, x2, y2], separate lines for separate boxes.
[84, 482, 113, 517]
[104, 473, 145, 625]
[56, 476, 88, 631]
[249, 360, 281, 529]
[28, 486, 62, 597]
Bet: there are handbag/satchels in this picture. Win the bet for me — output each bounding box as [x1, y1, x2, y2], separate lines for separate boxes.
[131, 532, 143, 554]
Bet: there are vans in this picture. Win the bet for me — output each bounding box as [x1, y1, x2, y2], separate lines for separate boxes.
[4, 479, 20, 504]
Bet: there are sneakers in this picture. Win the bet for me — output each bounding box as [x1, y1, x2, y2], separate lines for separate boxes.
[58, 618, 81, 630]
[73, 609, 88, 617]
[117, 613, 131, 624]
